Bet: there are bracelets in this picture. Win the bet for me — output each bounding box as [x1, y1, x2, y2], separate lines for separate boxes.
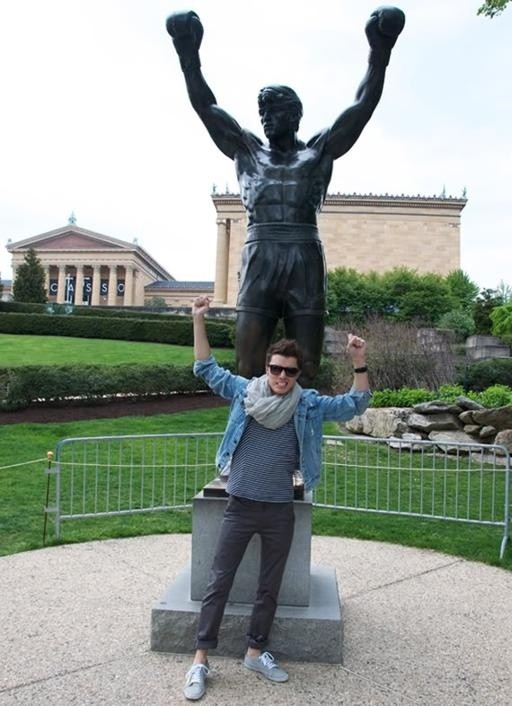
[353, 365, 368, 374]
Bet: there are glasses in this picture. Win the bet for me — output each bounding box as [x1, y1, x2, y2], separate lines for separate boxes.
[265, 364, 302, 378]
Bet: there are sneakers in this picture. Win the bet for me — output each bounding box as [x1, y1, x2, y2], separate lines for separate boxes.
[244, 652, 289, 683]
[183, 658, 210, 701]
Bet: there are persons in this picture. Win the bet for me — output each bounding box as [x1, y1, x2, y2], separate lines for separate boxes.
[181, 295, 372, 702]
[164, 3, 406, 483]
